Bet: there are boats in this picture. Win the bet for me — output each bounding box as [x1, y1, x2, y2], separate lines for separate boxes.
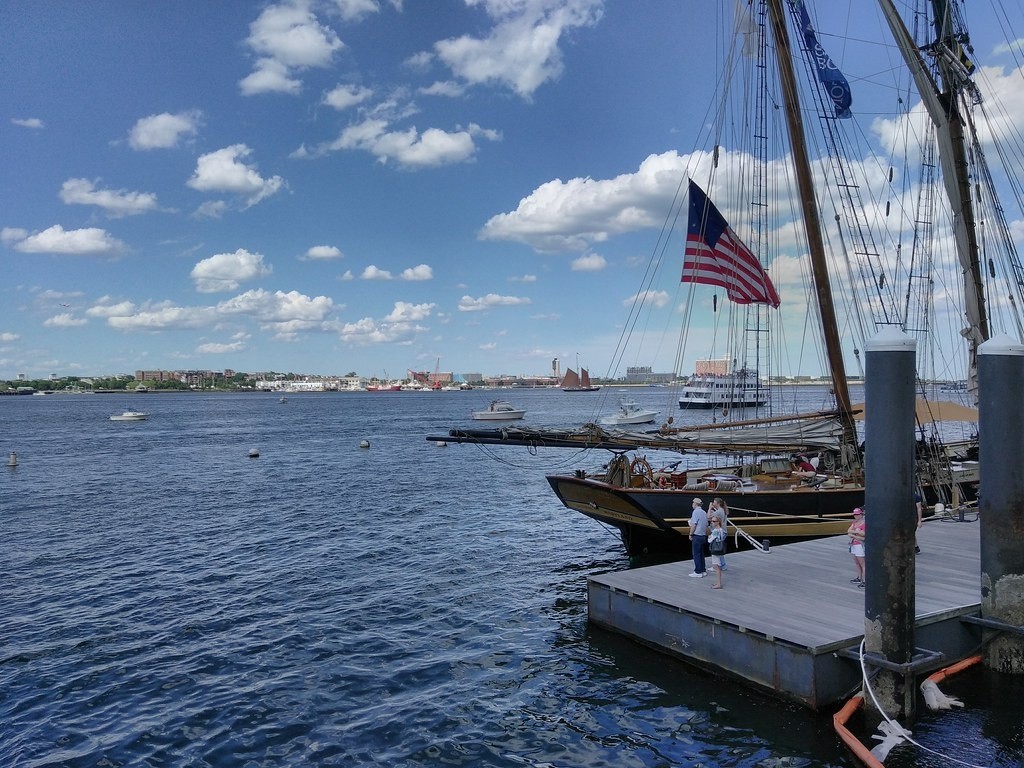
[678, 352, 771, 410]
[471, 397, 527, 419]
[110, 412, 150, 420]
[419, 388, 433, 392]
[366, 385, 402, 391]
[600, 394, 658, 425]
[441, 386, 461, 391]
[939, 381, 971, 394]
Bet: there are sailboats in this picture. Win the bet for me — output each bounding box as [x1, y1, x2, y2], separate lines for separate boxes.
[545, 0, 986, 556]
[559, 367, 600, 392]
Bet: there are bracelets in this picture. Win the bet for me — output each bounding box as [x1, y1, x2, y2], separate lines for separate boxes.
[689, 534, 693, 536]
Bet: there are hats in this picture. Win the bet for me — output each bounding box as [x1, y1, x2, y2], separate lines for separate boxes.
[693, 497, 704, 505]
[853, 507, 864, 514]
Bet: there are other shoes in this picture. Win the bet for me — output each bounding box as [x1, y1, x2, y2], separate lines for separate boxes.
[720, 566, 728, 571]
[707, 566, 718, 572]
[850, 577, 862, 583]
[711, 586, 722, 589]
[858, 581, 865, 588]
[712, 582, 717, 586]
[915, 546, 921, 553]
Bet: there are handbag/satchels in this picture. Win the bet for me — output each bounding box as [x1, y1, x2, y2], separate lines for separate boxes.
[848, 545, 851, 553]
[711, 531, 728, 555]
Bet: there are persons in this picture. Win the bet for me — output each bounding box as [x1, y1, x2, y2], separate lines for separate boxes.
[792, 456, 816, 491]
[705, 498, 728, 572]
[707, 515, 723, 589]
[915, 493, 923, 554]
[847, 508, 865, 589]
[688, 497, 708, 578]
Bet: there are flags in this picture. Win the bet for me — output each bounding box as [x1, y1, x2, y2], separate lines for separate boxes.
[681, 176, 780, 309]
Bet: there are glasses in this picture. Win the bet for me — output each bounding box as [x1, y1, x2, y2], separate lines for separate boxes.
[711, 520, 719, 523]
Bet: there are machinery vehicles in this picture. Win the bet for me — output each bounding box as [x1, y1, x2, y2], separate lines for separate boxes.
[408, 357, 441, 389]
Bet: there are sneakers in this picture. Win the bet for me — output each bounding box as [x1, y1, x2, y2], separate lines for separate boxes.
[702, 572, 708, 577]
[688, 571, 703, 578]
